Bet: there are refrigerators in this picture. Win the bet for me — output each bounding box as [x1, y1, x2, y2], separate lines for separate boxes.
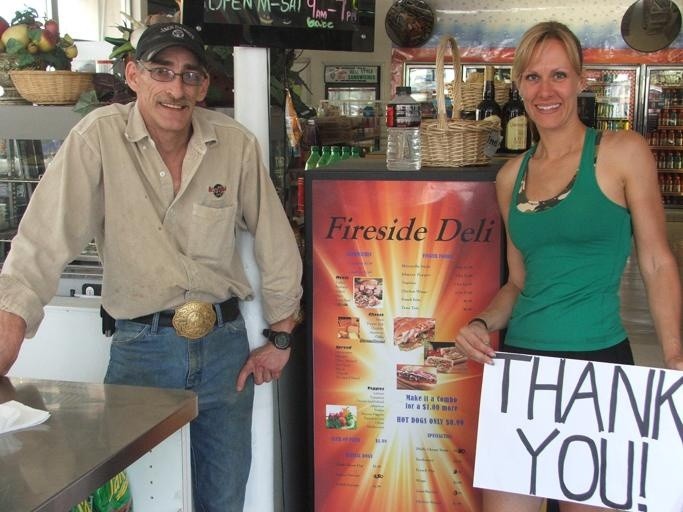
[322, 65, 380, 116]
[465, 64, 519, 124]
[573, 57, 641, 134]
[398, 61, 465, 121]
[642, 61, 682, 217]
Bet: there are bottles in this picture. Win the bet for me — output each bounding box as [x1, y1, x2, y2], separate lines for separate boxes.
[597, 103, 628, 129]
[502, 69, 529, 153]
[474, 65, 501, 155]
[644, 87, 683, 204]
[385, 86, 422, 171]
[286, 141, 305, 227]
[304, 146, 360, 171]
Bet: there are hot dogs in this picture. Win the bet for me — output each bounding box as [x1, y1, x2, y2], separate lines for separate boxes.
[394, 318, 468, 390]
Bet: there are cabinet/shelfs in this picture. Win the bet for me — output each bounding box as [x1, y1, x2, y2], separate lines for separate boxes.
[0, 105, 101, 279]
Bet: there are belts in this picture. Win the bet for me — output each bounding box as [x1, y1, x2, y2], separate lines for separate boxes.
[132, 299, 242, 338]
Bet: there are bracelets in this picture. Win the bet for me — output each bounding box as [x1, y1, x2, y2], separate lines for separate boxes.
[468, 318, 487, 330]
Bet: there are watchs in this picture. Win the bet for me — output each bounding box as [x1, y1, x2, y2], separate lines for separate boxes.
[263, 329, 296, 350]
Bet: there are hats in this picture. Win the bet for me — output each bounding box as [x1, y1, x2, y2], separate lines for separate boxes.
[134, 22, 212, 68]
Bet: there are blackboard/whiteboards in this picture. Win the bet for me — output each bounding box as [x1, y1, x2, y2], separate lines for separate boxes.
[181, 0, 374, 52]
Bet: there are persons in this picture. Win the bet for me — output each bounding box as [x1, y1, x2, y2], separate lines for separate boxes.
[0, 23, 304, 512]
[454, 21, 683, 512]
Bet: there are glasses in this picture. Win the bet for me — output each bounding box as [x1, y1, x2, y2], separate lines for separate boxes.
[141, 63, 204, 86]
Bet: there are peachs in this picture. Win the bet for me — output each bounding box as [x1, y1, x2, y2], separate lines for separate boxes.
[34, 29, 58, 53]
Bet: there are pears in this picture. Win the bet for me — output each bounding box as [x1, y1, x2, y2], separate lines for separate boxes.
[2, 23, 30, 49]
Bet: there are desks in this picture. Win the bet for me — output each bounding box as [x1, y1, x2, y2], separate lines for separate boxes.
[0, 376, 201, 512]
